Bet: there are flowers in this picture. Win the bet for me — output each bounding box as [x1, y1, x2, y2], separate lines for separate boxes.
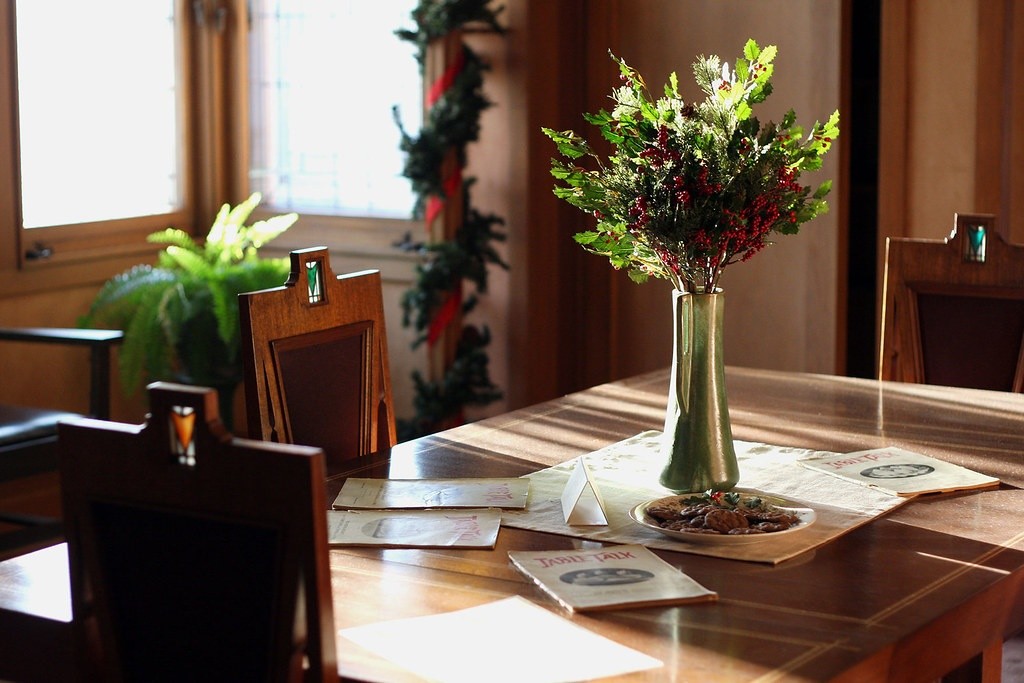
[541, 38, 842, 292]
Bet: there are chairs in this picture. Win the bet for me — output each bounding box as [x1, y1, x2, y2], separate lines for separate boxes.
[237, 246, 397, 469]
[57, 380, 341, 682]
[878, 211, 1024, 394]
[1, 325, 126, 534]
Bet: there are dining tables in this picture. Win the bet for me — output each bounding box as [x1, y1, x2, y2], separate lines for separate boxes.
[0, 365, 1024, 682]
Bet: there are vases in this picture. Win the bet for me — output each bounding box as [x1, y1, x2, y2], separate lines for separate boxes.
[657, 286, 739, 496]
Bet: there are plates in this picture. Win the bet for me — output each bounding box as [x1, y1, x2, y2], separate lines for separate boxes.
[629, 494, 816, 545]
